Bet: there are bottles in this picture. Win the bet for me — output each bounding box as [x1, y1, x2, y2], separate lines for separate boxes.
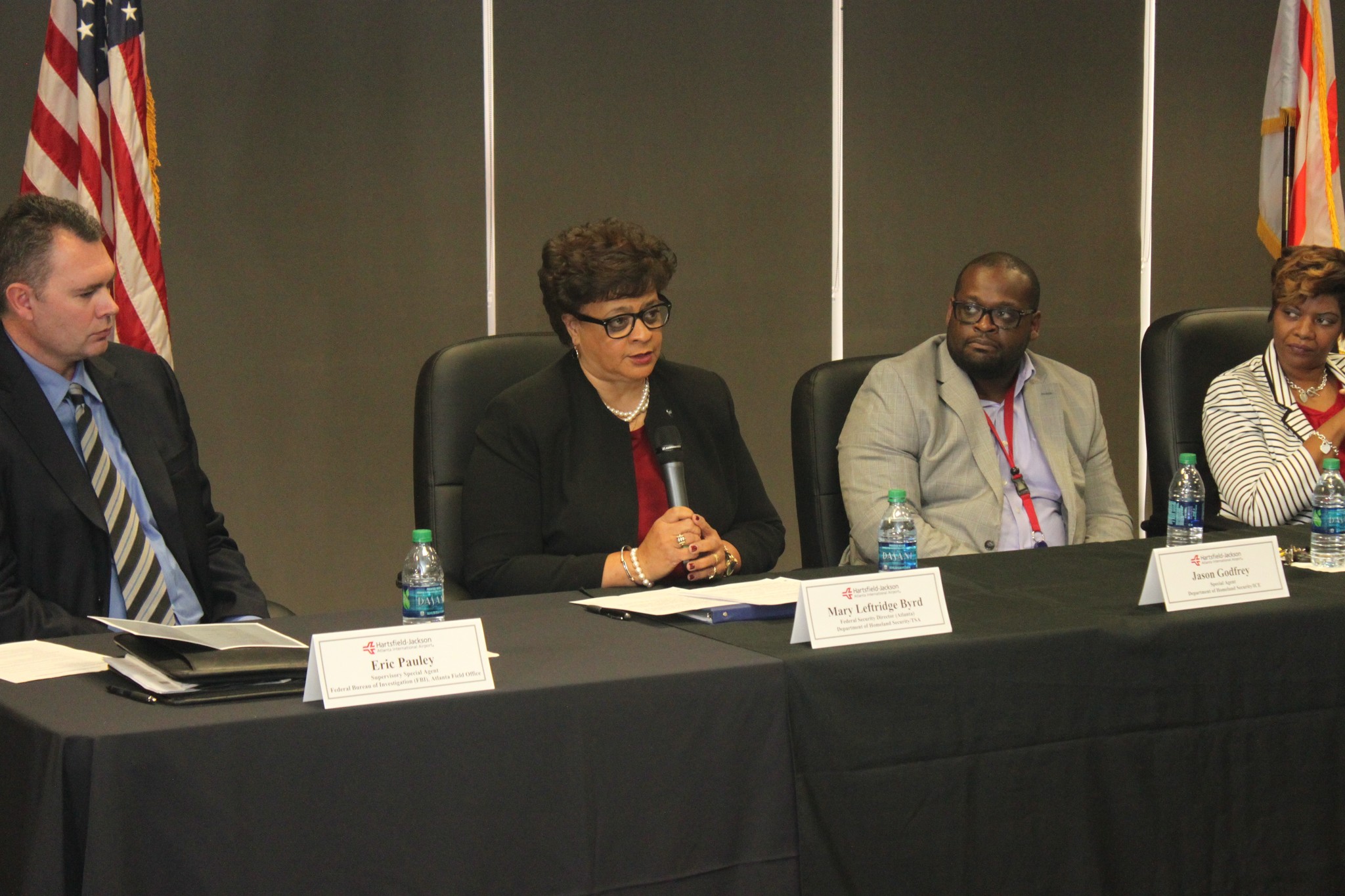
[1165, 453, 1204, 551]
[1310, 457, 1345, 569]
[403, 528, 446, 629]
[878, 490, 917, 578]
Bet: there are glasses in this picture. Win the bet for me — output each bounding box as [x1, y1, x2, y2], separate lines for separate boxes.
[569, 293, 672, 340]
[952, 299, 1035, 330]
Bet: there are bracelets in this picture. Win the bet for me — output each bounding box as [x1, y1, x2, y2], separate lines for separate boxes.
[629, 547, 655, 589]
[1308, 430, 1339, 458]
[620, 545, 641, 586]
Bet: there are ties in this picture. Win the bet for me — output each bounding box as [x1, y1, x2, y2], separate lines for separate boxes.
[66, 383, 177, 627]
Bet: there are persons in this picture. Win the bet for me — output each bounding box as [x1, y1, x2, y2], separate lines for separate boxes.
[837, 250, 1134, 567]
[1201, 244, 1345, 533]
[0, 194, 270, 644]
[461, 215, 788, 600]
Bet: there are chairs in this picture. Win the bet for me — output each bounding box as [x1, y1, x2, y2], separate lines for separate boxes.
[1142, 308, 1273, 538]
[793, 350, 903, 562]
[408, 335, 573, 598]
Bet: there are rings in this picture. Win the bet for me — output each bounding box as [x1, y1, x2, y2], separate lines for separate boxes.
[676, 533, 686, 547]
[708, 566, 717, 580]
[711, 553, 721, 566]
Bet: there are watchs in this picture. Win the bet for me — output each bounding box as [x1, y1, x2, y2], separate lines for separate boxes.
[721, 545, 738, 578]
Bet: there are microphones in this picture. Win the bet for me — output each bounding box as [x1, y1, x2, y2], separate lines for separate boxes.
[653, 424, 705, 575]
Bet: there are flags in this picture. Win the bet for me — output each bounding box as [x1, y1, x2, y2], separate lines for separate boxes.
[1255, 1, 1345, 357]
[16, 0, 176, 376]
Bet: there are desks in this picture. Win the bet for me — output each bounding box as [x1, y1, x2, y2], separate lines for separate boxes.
[0, 506, 1343, 893]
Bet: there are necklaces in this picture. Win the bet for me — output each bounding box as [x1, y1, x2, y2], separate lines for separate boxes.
[1283, 367, 1327, 403]
[602, 375, 650, 424]
